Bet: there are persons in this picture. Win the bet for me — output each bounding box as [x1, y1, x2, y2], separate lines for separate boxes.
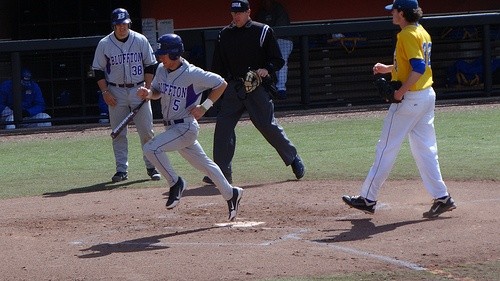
[0, 69, 52, 130]
[432, 54, 500, 98]
[447, 23, 500, 41]
[136, 34, 243, 222]
[343, 0, 455, 219]
[257, 0, 295, 93]
[92, 8, 161, 181]
[97, 91, 110, 124]
[203, 0, 304, 185]
[45, 64, 73, 125]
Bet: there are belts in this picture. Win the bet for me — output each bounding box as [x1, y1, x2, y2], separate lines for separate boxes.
[163, 119, 184, 126]
[107, 80, 144, 87]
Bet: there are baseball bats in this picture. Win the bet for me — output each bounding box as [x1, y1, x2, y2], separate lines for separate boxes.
[110, 99, 146, 138]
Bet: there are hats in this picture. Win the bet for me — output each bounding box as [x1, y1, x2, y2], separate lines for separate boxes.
[230, 0, 250, 12]
[385, 0, 419, 10]
[20, 71, 33, 87]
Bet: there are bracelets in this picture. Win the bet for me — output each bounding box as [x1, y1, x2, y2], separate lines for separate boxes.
[145, 89, 153, 99]
[201, 98, 213, 110]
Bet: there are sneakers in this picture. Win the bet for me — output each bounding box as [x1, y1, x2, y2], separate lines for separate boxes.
[290, 154, 305, 179]
[342, 195, 376, 214]
[423, 193, 456, 219]
[147, 168, 160, 181]
[226, 186, 244, 220]
[202, 176, 232, 184]
[112, 172, 128, 182]
[166, 176, 186, 209]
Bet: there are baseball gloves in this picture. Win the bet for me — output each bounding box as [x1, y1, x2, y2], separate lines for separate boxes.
[240, 67, 263, 94]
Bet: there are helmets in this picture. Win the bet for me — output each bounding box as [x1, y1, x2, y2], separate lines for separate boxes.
[111, 8, 132, 24]
[152, 34, 184, 55]
